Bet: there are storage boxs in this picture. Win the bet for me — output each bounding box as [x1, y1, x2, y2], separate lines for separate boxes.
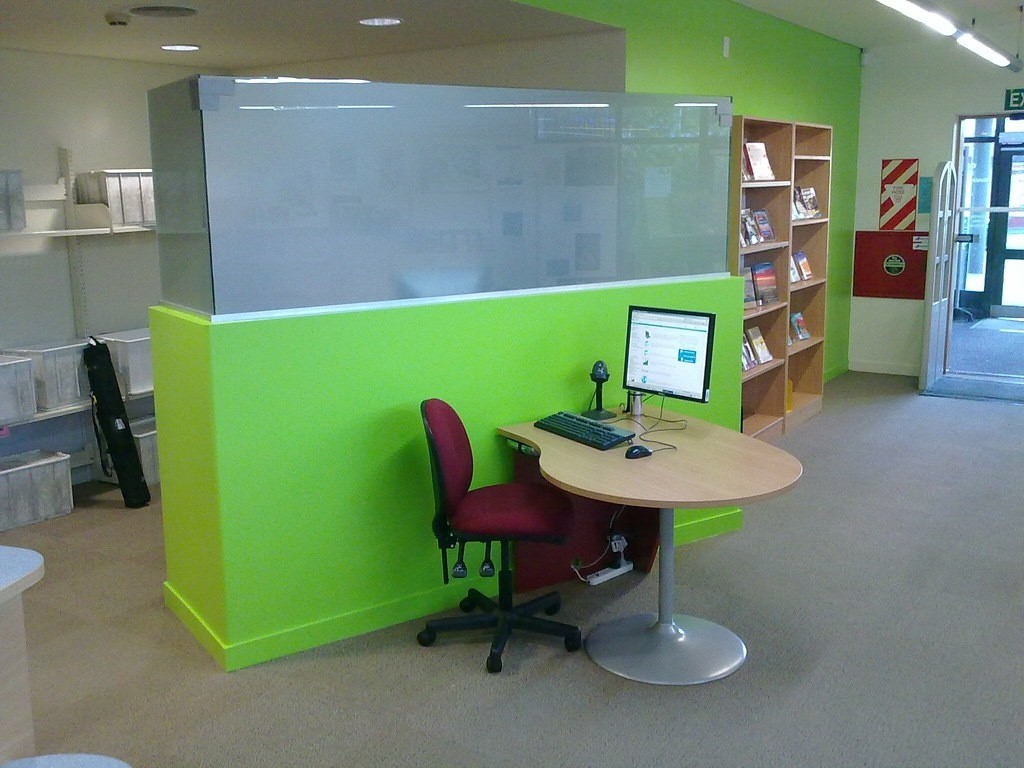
[77, 168, 157, 228]
[0, 339, 105, 412]
[0, 448, 74, 533]
[97, 327, 154, 395]
[100, 416, 160, 487]
[0, 355, 35, 425]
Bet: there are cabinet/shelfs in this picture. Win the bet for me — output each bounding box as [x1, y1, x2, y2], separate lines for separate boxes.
[727, 115, 833, 444]
[0, 225, 157, 432]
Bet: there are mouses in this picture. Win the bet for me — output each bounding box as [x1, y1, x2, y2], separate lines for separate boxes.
[625, 446, 653, 459]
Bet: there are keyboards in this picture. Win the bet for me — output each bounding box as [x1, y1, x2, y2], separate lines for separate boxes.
[534, 411, 636, 451]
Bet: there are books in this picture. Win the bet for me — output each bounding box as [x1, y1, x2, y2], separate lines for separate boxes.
[790, 256, 801, 282]
[793, 250, 814, 281]
[740, 255, 777, 309]
[792, 185, 821, 220]
[742, 326, 773, 372]
[740, 207, 775, 249]
[790, 313, 811, 339]
[743, 139, 776, 180]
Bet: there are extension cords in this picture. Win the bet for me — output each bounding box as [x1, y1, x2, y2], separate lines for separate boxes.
[586, 562, 633, 586]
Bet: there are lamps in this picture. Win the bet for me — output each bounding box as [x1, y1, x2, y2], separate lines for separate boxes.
[876, 0, 1023, 72]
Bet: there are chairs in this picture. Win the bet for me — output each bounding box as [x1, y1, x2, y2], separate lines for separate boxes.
[417, 398, 582, 673]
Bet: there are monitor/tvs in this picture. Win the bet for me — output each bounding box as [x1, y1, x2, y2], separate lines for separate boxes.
[623, 305, 716, 403]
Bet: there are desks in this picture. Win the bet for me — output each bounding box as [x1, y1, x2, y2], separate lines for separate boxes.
[497, 401, 803, 686]
